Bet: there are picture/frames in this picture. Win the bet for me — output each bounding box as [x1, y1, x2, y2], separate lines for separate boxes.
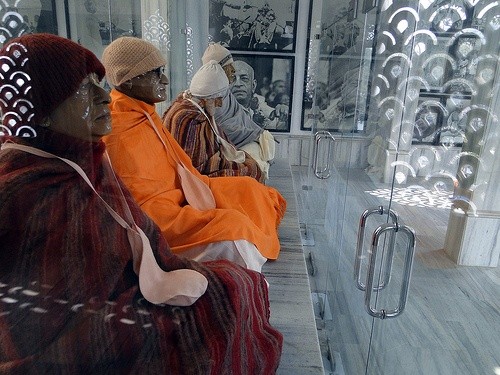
[229, 54, 295, 132]
[209, 0, 299, 53]
[300, 0, 381, 133]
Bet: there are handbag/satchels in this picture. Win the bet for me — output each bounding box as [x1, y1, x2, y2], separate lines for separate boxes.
[217, 134, 245, 164]
[126, 223, 208, 306]
[175, 161, 217, 212]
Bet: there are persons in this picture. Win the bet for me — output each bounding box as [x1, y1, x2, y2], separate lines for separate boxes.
[231, 59, 371, 133]
[0, 32, 283, 375]
[102, 37, 286, 288]
[161, 60, 266, 187]
[201, 42, 276, 180]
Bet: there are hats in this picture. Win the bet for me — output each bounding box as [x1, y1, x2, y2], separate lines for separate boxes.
[201, 43, 233, 69]
[190, 61, 229, 97]
[0, 33, 106, 137]
[102, 37, 165, 86]
